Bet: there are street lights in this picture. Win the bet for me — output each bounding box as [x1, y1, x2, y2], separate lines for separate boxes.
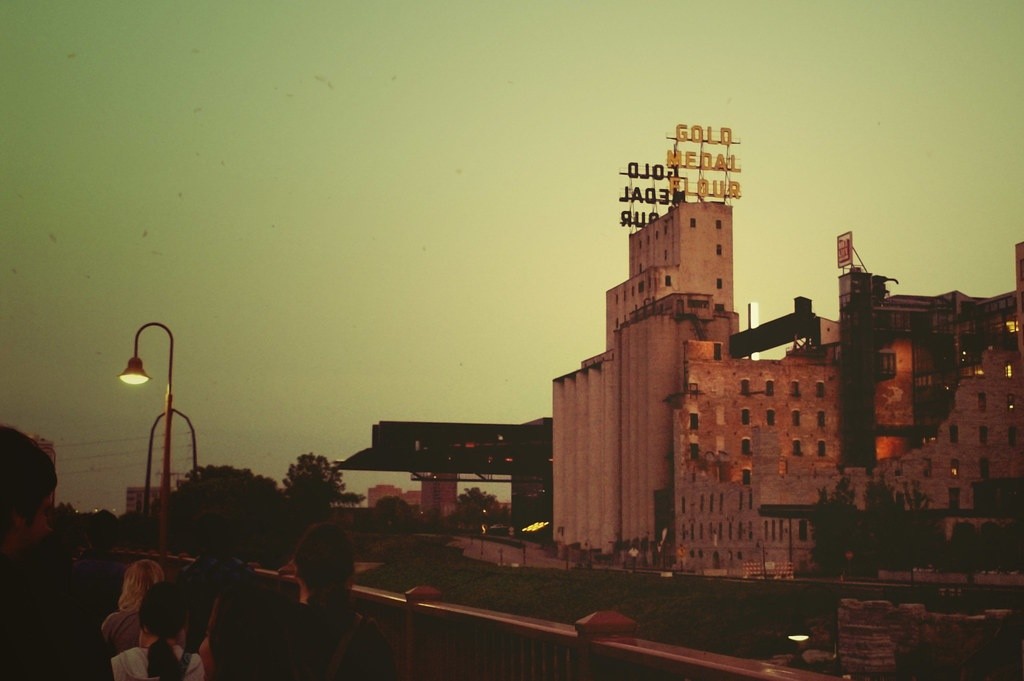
[116, 322, 173, 552]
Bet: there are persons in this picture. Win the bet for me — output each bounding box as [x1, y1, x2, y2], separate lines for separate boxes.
[0, 424, 115, 681]
[174, 513, 266, 653]
[110, 581, 206, 681]
[230, 520, 398, 681]
[102, 558, 187, 653]
